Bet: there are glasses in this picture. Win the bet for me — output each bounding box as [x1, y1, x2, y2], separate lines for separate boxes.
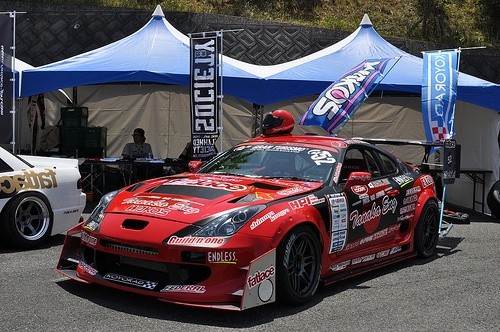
[132, 134, 140, 136]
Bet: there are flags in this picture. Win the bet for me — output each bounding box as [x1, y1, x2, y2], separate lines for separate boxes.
[189, 33, 220, 160]
[0, 13, 13, 144]
[422, 50, 458, 152]
[297, 58, 391, 135]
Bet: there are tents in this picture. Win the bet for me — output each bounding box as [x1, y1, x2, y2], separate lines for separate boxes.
[0, 3, 500, 216]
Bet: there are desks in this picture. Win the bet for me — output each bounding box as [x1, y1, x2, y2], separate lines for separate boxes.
[427, 167, 494, 216]
[81, 159, 187, 196]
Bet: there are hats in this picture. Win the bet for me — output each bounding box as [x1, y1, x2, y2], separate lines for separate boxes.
[134, 128, 145, 136]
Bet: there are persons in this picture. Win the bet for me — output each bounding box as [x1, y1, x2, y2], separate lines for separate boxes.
[252, 109, 296, 177]
[121, 128, 153, 158]
[178, 142, 219, 159]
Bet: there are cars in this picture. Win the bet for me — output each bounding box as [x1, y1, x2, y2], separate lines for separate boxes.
[56, 134, 443, 314]
[0, 146, 87, 249]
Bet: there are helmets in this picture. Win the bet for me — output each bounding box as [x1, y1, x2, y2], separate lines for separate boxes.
[261, 110, 296, 135]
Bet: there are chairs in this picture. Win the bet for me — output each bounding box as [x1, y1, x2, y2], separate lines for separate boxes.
[265, 152, 298, 174]
[340, 159, 367, 178]
